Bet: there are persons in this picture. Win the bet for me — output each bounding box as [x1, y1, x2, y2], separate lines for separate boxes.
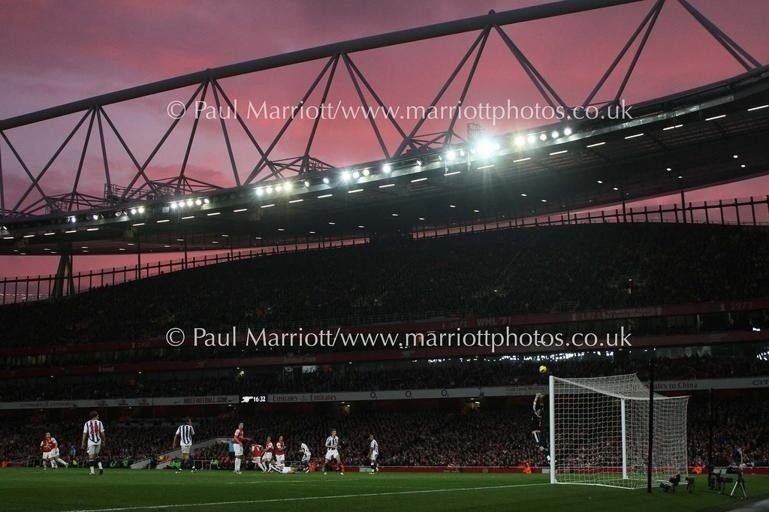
[2, 223, 769, 476]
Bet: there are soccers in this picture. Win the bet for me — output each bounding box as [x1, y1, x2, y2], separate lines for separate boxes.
[539, 366, 547, 373]
